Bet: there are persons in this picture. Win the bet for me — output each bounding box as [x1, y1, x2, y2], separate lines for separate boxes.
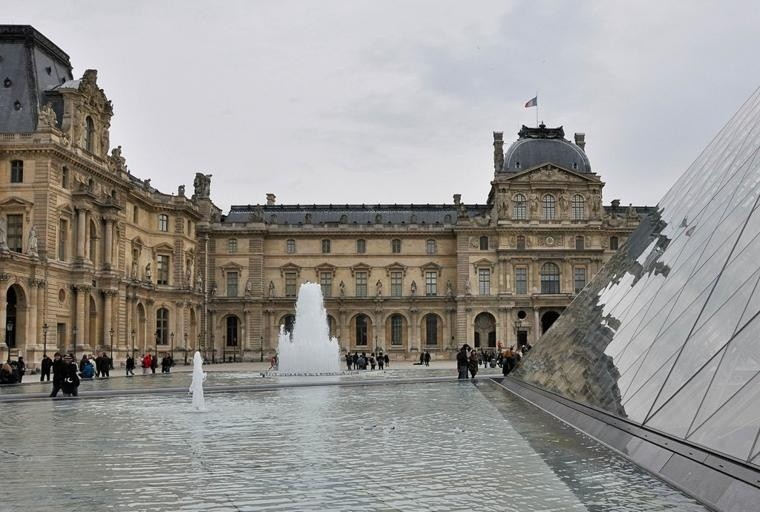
[455, 343, 533, 379]
[345, 351, 390, 371]
[141, 353, 173, 375]
[40, 354, 52, 381]
[63, 353, 80, 396]
[271, 356, 276, 368]
[126, 355, 135, 376]
[48, 352, 65, 397]
[420, 351, 425, 364]
[0, 355, 25, 384]
[425, 351, 431, 366]
[69, 351, 113, 378]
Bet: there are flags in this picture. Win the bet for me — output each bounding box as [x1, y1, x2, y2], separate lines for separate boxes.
[524, 96, 537, 107]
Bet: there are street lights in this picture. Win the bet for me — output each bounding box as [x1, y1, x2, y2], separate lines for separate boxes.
[514, 320, 521, 349]
[6, 320, 264, 370]
